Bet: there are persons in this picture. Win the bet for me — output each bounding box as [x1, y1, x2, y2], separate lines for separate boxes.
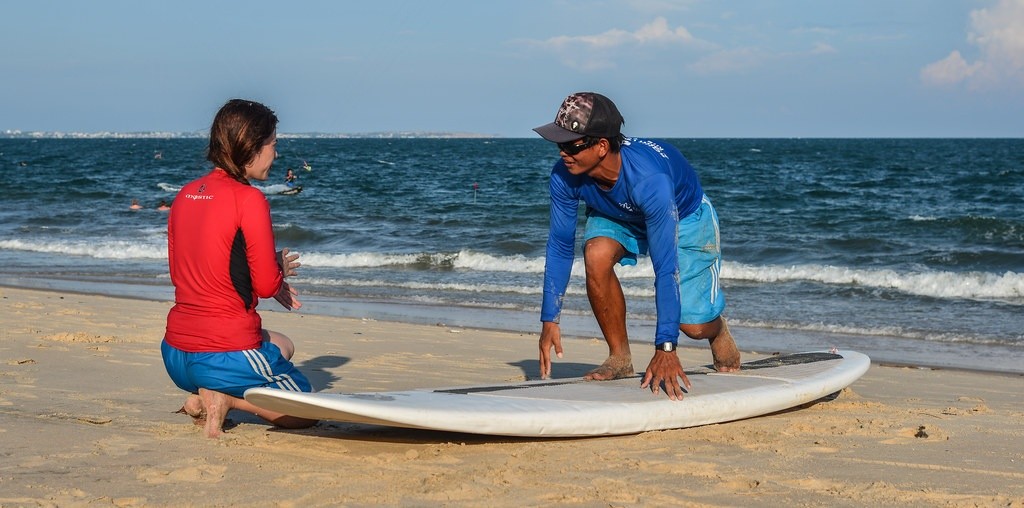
[532, 92, 741, 401]
[161, 99, 320, 439]
[129, 200, 142, 211]
[157, 201, 171, 211]
[284, 168, 298, 186]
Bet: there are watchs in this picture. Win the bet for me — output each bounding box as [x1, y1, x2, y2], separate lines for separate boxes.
[655, 341, 678, 352]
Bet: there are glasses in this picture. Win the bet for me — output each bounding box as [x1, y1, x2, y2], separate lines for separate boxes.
[557, 139, 592, 157]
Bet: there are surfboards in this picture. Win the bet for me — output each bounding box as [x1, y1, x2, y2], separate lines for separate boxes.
[242, 347, 871, 438]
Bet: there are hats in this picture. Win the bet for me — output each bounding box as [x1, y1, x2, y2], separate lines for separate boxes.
[533, 92, 625, 143]
